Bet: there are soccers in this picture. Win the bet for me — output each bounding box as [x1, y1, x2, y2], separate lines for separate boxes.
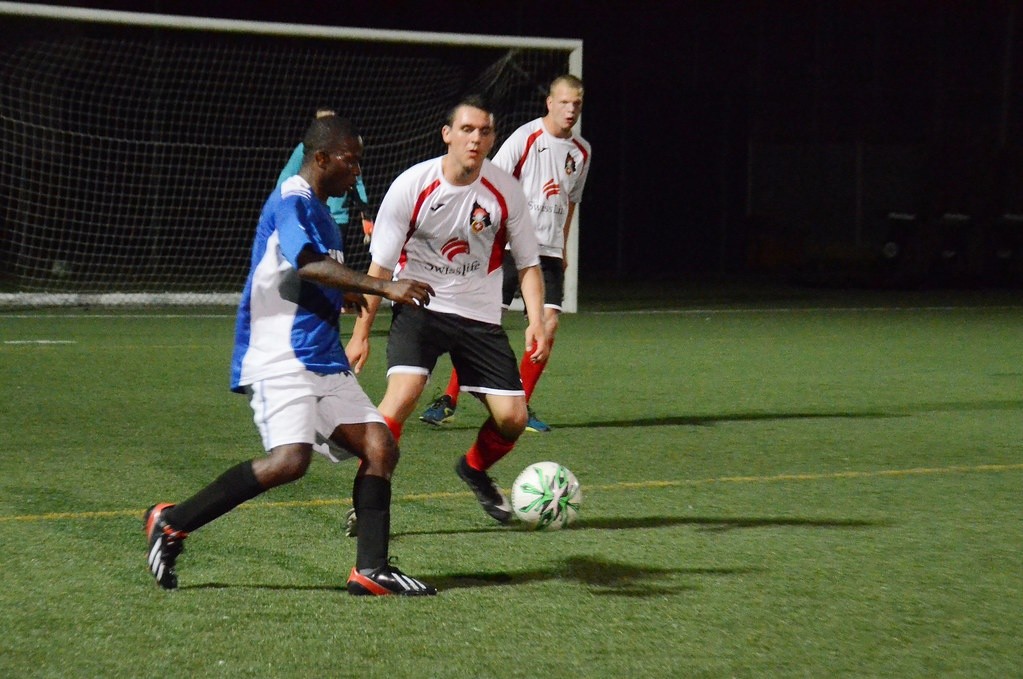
[511, 461, 582, 532]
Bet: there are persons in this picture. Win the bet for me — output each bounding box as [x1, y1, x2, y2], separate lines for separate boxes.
[143, 114, 435, 598]
[344, 90, 550, 536]
[276, 100, 374, 250]
[419, 75, 593, 432]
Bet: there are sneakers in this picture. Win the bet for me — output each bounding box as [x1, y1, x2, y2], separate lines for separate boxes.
[143, 503, 187, 589]
[339, 508, 359, 537]
[525, 405, 551, 432]
[346, 556, 438, 596]
[419, 387, 456, 426]
[456, 456, 512, 523]
